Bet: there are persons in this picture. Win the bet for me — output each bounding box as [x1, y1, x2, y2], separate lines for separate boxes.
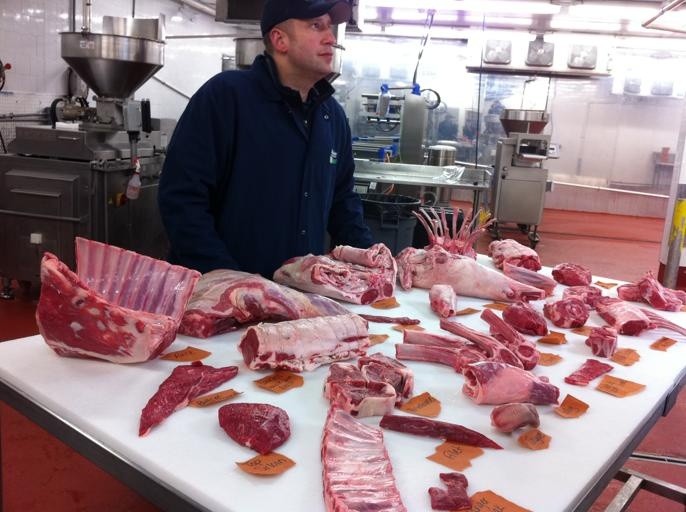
[487, 99, 506, 115]
[156, 0, 375, 280]
[463, 117, 477, 143]
[438, 114, 458, 141]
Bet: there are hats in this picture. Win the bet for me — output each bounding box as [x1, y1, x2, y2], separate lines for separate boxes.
[261, 0, 352, 36]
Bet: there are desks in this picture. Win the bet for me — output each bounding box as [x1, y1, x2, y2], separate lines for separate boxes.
[1, 253, 686, 512]
[353, 156, 494, 252]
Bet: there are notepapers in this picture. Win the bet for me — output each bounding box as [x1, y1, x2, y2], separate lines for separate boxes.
[236, 449, 296, 476]
[611, 348, 640, 365]
[189, 388, 244, 409]
[423, 439, 485, 472]
[457, 306, 482, 316]
[466, 490, 534, 512]
[158, 345, 212, 361]
[598, 375, 646, 401]
[552, 393, 589, 417]
[595, 280, 618, 288]
[253, 368, 306, 395]
[399, 391, 441, 418]
[485, 300, 509, 312]
[650, 336, 678, 350]
[536, 353, 563, 367]
[571, 324, 599, 336]
[370, 333, 389, 345]
[537, 328, 568, 344]
[519, 429, 552, 452]
[369, 296, 400, 308]
[393, 324, 426, 335]
